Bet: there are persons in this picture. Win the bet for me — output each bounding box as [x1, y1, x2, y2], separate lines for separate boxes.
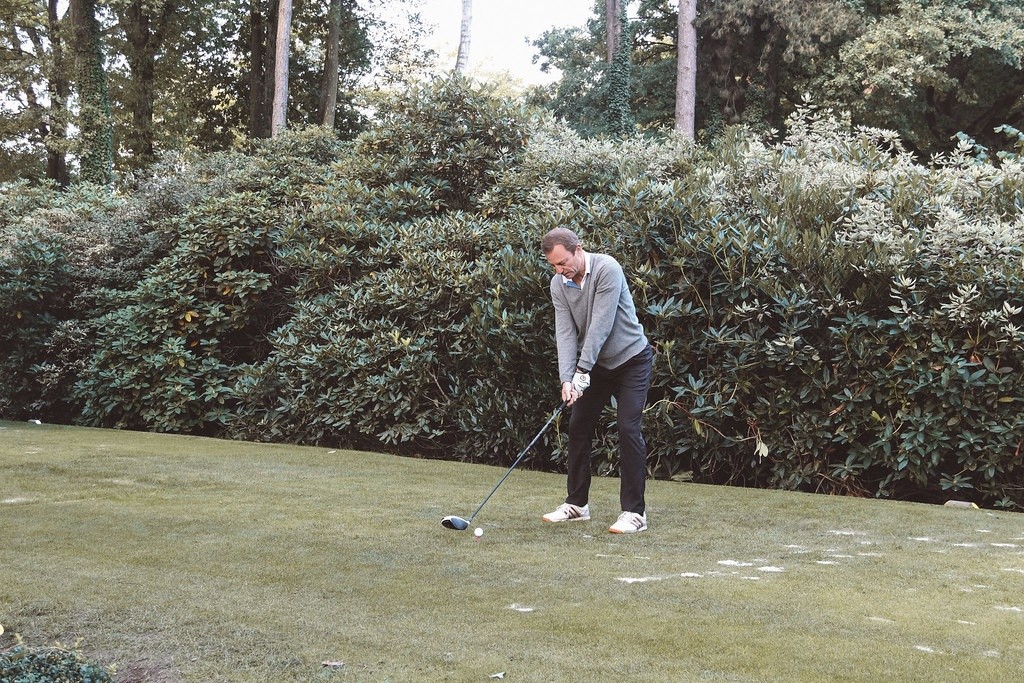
[541, 227, 653, 534]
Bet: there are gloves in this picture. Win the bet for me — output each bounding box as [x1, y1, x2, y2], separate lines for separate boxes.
[572, 367, 590, 398]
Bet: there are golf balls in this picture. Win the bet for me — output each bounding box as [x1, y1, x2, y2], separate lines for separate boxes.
[474, 528, 483, 536]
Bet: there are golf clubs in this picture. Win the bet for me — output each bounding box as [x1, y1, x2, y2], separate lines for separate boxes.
[440, 388, 573, 531]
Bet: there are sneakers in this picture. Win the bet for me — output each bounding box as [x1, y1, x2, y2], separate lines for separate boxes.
[542, 504, 591, 524]
[609, 511, 647, 533]
